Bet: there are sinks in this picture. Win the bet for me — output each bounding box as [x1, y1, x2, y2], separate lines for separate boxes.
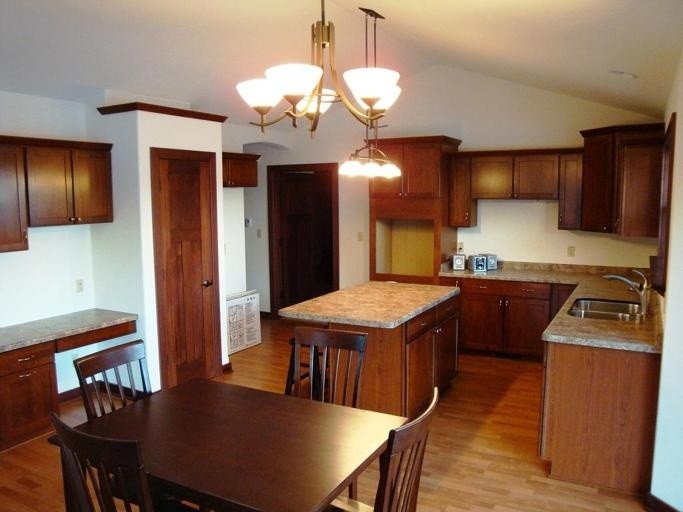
[565, 297, 641, 322]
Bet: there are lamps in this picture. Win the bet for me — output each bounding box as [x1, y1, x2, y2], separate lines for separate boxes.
[338, 5, 402, 176]
[233, 0, 402, 180]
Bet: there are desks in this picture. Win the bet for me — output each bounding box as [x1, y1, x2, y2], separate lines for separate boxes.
[0, 306, 137, 451]
[277, 279, 461, 421]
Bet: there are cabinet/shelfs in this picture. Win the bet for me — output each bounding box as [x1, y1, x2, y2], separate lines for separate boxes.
[579, 125, 615, 240]
[439, 274, 459, 289]
[471, 150, 559, 201]
[460, 279, 550, 360]
[24, 137, 114, 229]
[222, 151, 261, 187]
[616, 123, 665, 241]
[365, 135, 463, 200]
[369, 200, 457, 282]
[555, 150, 582, 231]
[449, 153, 476, 227]
[0, 134, 28, 253]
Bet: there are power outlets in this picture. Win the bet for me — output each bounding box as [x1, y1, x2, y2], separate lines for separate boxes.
[457, 242, 464, 253]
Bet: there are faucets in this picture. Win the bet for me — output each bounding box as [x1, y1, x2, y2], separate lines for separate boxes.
[601, 269, 649, 316]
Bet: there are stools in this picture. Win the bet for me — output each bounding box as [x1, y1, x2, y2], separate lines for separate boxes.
[284, 335, 329, 400]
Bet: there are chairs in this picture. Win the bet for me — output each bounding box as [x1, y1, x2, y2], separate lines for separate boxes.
[322, 387, 439, 512]
[73, 339, 176, 512]
[292, 327, 369, 502]
[48, 411, 199, 512]
